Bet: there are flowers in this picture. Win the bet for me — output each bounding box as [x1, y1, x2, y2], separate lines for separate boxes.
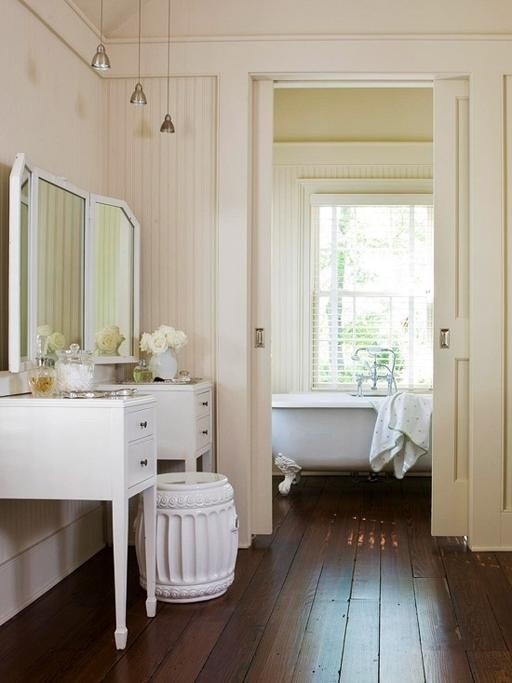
[29, 325, 65, 369]
[92, 325, 125, 358]
[139, 324, 188, 355]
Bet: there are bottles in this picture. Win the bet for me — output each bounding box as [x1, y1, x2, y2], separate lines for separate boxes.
[52, 342, 97, 397]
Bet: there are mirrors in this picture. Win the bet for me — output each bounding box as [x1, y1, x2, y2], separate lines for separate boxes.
[7, 153, 142, 373]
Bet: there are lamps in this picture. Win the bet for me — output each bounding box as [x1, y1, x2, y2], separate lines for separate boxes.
[90, 1, 175, 134]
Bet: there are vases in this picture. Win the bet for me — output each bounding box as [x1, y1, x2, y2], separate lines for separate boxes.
[148, 349, 180, 381]
[27, 363, 55, 395]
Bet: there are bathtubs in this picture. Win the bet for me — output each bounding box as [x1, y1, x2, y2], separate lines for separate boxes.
[272, 393, 432, 496]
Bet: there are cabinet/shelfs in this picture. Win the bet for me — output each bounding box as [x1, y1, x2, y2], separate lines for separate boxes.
[98, 381, 215, 474]
[2, 394, 159, 649]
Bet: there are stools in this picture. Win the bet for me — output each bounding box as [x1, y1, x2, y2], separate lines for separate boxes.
[133, 471, 239, 605]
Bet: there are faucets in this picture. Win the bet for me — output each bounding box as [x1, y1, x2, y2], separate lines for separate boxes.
[371, 366, 378, 390]
